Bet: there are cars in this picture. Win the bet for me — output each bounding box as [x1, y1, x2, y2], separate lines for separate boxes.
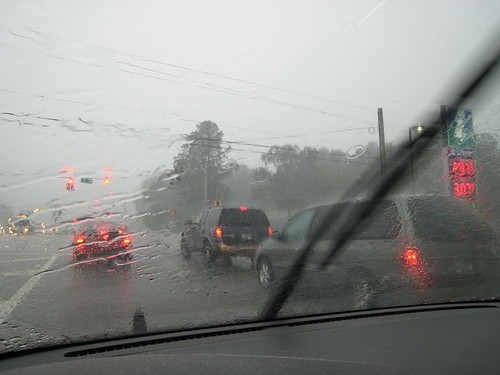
[74, 221, 134, 272]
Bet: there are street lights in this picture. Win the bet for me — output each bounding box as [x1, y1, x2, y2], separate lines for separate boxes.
[408, 126, 426, 178]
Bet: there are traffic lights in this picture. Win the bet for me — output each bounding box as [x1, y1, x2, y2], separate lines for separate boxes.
[65, 178, 74, 191]
[105, 177, 109, 183]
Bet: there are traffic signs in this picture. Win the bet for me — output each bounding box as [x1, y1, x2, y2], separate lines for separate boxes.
[447, 127, 474, 149]
[447, 106, 473, 128]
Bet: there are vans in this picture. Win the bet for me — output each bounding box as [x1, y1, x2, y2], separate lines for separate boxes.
[251, 193, 499, 311]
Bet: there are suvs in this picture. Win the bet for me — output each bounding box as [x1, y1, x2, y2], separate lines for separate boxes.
[180, 204, 275, 263]
